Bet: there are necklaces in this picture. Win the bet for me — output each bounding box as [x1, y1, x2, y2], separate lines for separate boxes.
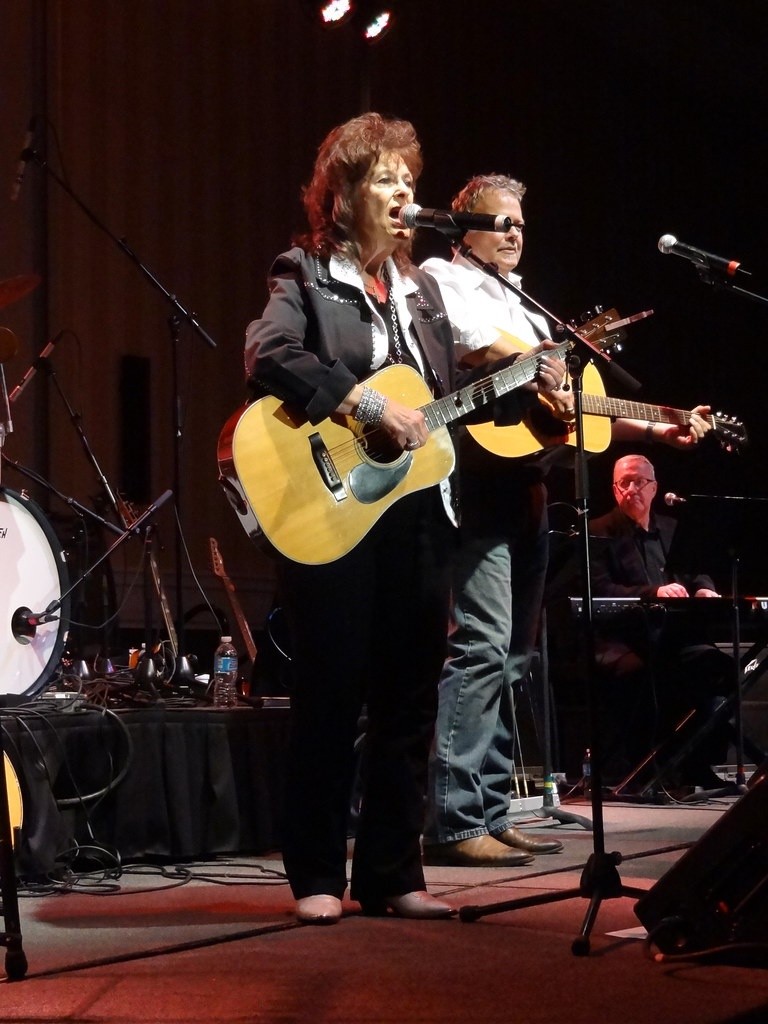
[364, 283, 402, 365]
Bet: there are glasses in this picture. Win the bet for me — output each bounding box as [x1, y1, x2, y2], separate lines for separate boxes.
[614, 477, 653, 489]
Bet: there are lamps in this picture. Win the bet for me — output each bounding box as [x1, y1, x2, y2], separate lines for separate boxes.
[301, 0, 392, 39]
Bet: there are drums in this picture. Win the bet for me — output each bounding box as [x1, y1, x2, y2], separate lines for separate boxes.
[0, 487, 70, 702]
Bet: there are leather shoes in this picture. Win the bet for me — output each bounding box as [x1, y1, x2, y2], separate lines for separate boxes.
[357, 891, 462, 917]
[423, 832, 541, 869]
[510, 827, 567, 855]
[294, 893, 346, 924]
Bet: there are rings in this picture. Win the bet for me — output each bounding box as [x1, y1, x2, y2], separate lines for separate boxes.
[407, 440, 419, 448]
[566, 407, 574, 412]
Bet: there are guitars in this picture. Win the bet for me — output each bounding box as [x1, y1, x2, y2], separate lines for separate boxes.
[217, 306, 624, 564]
[465, 357, 747, 466]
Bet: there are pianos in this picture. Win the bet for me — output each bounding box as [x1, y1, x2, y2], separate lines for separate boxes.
[560, 597, 768, 787]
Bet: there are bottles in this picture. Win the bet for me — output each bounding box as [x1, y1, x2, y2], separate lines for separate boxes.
[214, 637, 238, 708]
[582, 747, 592, 799]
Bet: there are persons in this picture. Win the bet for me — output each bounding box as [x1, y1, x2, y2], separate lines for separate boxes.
[418, 175, 711, 867]
[243, 113, 563, 923]
[587, 455, 739, 790]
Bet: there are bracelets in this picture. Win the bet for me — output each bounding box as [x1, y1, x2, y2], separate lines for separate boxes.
[644, 422, 656, 440]
[353, 385, 388, 426]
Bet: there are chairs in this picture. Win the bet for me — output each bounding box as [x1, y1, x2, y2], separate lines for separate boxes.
[545, 502, 677, 799]
